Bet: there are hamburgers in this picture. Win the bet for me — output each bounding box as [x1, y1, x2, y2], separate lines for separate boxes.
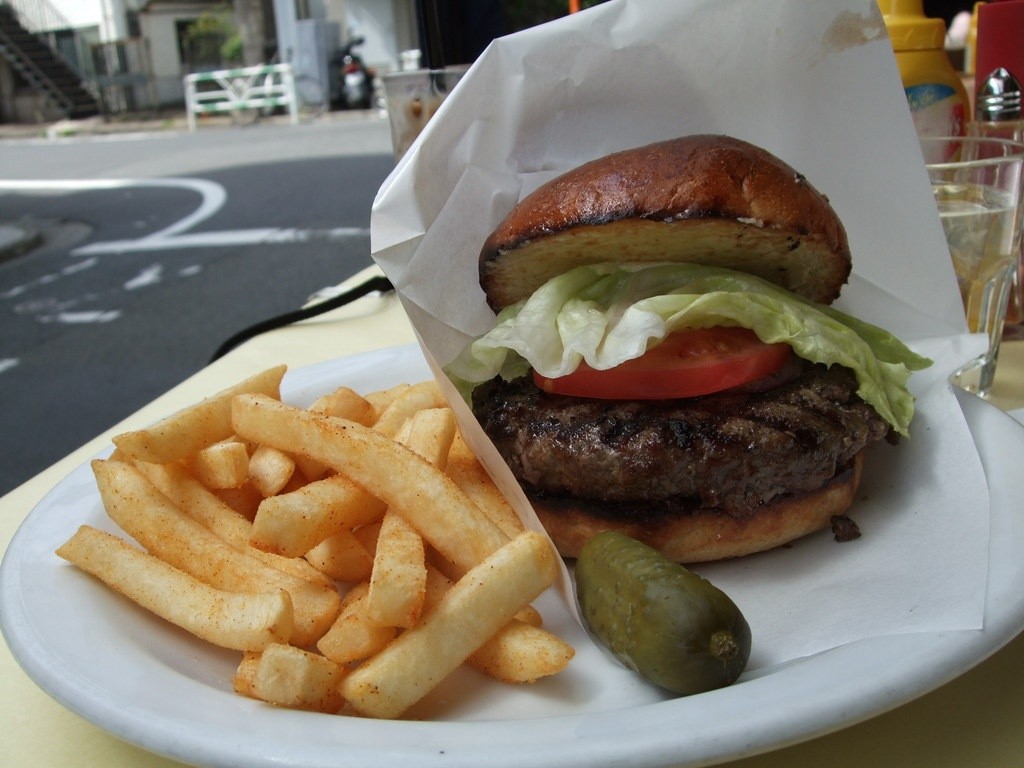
[466, 134, 928, 562]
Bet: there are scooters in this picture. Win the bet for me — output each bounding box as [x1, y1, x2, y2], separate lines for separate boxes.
[327, 37, 373, 109]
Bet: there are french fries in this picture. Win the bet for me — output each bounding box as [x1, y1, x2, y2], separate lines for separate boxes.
[59, 365, 571, 718]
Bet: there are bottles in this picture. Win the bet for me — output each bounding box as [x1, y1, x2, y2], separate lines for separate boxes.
[868, 0, 972, 198]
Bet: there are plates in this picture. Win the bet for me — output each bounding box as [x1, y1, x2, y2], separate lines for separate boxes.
[0, 340, 1024, 768]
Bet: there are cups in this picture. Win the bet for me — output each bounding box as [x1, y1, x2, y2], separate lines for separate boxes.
[381, 67, 471, 163]
[904, 134, 1024, 400]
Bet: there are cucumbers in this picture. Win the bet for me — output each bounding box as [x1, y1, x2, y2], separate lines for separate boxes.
[576, 535, 751, 694]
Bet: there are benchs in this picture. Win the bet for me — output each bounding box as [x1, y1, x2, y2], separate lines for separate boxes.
[181, 62, 300, 130]
